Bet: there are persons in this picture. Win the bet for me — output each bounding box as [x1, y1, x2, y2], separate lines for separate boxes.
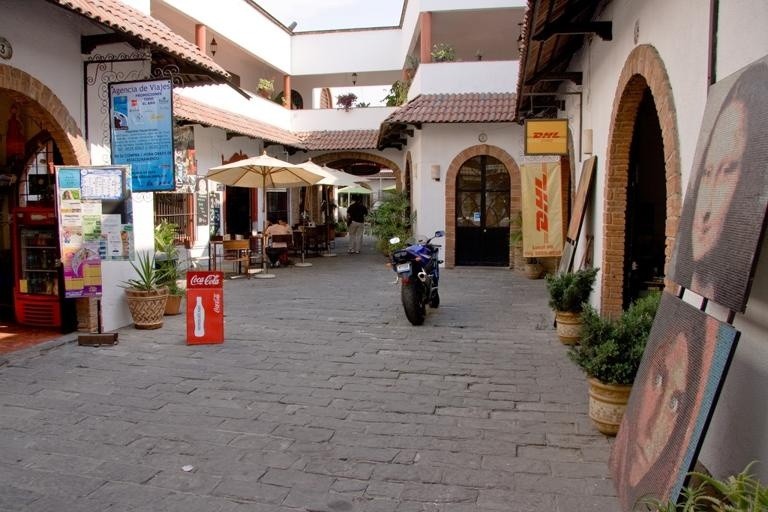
[671, 63, 768, 313]
[608, 291, 708, 512]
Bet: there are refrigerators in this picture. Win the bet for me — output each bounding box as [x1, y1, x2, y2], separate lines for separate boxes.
[10, 205, 60, 328]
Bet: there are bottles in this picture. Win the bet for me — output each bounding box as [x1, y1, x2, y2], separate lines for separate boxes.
[22, 228, 59, 295]
[193, 296, 205, 337]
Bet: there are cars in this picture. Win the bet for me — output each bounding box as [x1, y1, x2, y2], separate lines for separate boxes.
[369, 200, 387, 213]
[497, 217, 509, 226]
[456, 217, 478, 227]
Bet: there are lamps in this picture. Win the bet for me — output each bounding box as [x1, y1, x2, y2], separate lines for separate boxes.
[207, 35, 218, 56]
[350, 73, 360, 86]
[430, 164, 441, 182]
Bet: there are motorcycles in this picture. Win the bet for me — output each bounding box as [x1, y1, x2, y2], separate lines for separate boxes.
[389, 229, 447, 325]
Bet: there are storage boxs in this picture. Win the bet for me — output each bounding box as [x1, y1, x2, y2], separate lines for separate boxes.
[184, 269, 226, 346]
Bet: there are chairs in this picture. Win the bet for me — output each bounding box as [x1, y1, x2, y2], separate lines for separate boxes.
[209, 223, 336, 279]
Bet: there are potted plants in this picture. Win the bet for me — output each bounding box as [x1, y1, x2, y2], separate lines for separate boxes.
[116, 249, 179, 329]
[564, 291, 664, 437]
[153, 216, 184, 316]
[508, 210, 544, 280]
[544, 265, 601, 346]
[336, 222, 349, 238]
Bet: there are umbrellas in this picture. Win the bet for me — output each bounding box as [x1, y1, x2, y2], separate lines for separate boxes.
[207, 150, 396, 272]
